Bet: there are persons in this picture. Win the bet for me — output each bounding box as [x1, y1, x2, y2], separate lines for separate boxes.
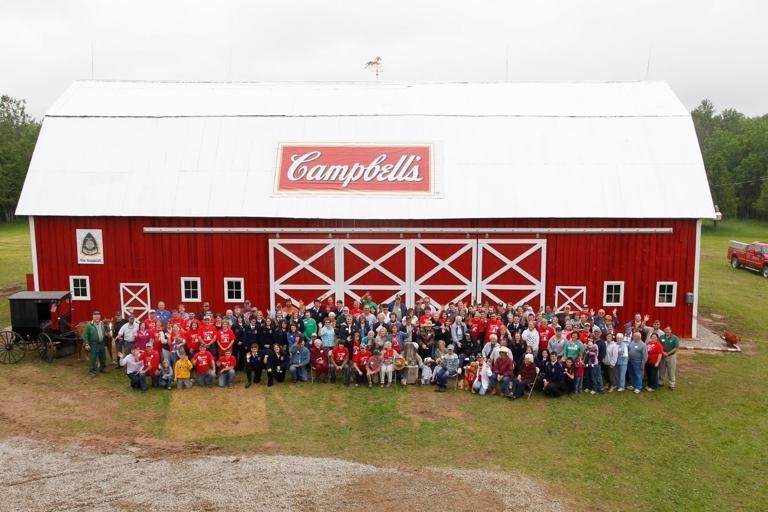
[84, 292, 679, 399]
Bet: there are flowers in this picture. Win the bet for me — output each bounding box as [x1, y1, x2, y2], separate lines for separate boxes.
[720, 331, 742, 347]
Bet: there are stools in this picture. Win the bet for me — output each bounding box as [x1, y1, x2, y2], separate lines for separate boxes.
[383, 370, 397, 385]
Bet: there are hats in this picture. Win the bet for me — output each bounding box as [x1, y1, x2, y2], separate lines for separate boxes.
[421, 321, 435, 327]
[448, 344, 455, 350]
[497, 346, 509, 353]
[395, 358, 405, 370]
[424, 357, 434, 364]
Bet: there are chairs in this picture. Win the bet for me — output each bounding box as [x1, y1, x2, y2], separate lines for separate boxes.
[310, 358, 330, 384]
[525, 367, 540, 400]
[448, 356, 462, 391]
[327, 349, 350, 382]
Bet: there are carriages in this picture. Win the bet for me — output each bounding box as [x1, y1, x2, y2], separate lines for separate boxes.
[0, 290, 132, 367]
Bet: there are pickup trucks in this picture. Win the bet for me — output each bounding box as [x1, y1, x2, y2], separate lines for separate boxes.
[727, 239, 768, 277]
